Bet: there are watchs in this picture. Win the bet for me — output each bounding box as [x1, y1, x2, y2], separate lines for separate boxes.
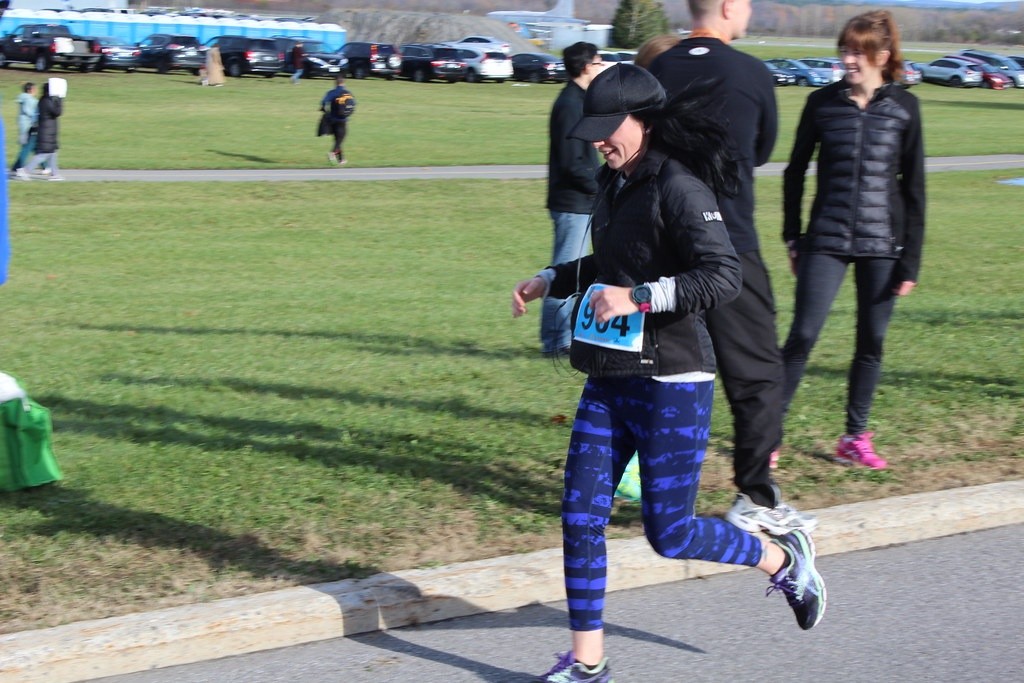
[631, 283, 652, 312]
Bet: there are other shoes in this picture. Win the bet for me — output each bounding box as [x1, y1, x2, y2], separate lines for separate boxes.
[11, 166, 63, 181]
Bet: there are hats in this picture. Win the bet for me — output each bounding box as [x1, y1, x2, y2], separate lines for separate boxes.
[566, 62, 666, 140]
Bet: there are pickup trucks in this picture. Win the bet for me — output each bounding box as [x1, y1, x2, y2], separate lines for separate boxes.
[0, 24, 103, 72]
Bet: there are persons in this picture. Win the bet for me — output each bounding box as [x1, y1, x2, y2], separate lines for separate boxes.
[10, 82, 51, 173]
[540, 42, 602, 357]
[646, 0, 817, 536]
[635, 35, 679, 69]
[321, 75, 352, 164]
[511, 64, 828, 683]
[290, 42, 307, 84]
[769, 10, 927, 465]
[17, 82, 64, 181]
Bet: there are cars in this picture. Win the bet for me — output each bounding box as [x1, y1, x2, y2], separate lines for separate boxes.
[458, 45, 514, 83]
[96, 37, 143, 73]
[133, 33, 208, 75]
[945, 55, 1015, 90]
[798, 57, 846, 83]
[45, 7, 303, 24]
[512, 52, 568, 84]
[762, 58, 830, 87]
[200, 35, 285, 78]
[913, 58, 983, 88]
[598, 50, 639, 70]
[960, 48, 1024, 88]
[903, 60, 921, 89]
[443, 36, 511, 56]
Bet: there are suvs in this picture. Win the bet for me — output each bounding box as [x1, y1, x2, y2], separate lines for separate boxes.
[336, 42, 402, 81]
[270, 35, 349, 77]
[400, 42, 467, 84]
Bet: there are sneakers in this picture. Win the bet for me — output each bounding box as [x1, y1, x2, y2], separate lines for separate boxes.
[764, 529, 828, 631]
[725, 483, 819, 537]
[770, 447, 780, 469]
[530, 651, 611, 683]
[832, 435, 885, 470]
